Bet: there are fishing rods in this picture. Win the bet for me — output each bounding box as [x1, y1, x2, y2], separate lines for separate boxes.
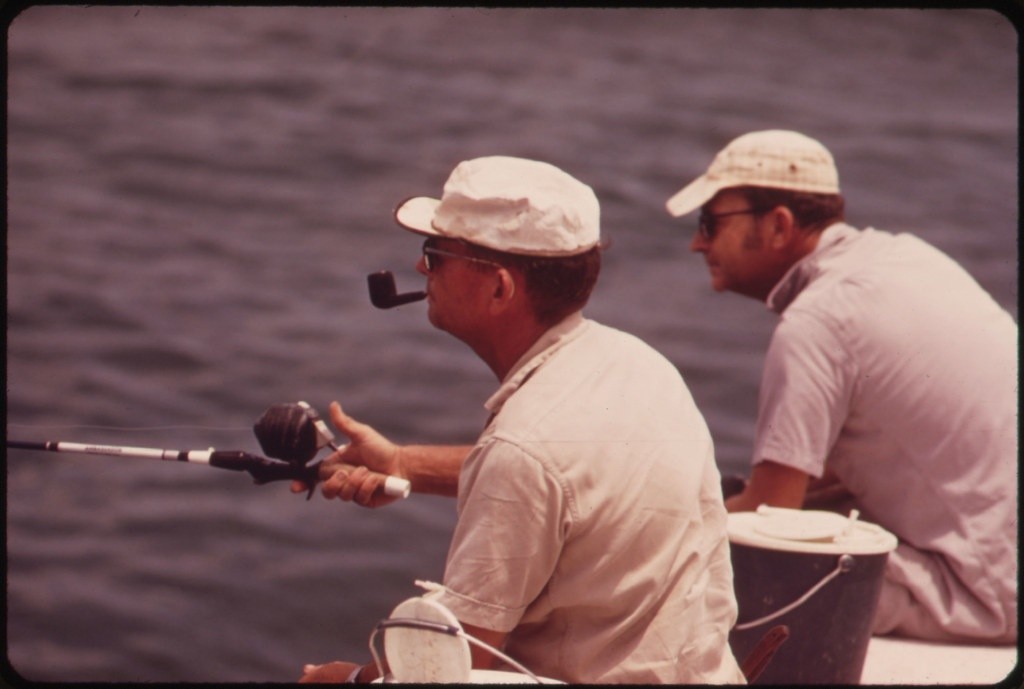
[7, 401, 412, 500]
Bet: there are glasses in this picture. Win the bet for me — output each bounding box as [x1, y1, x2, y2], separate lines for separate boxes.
[698, 206, 769, 241]
[422, 238, 501, 272]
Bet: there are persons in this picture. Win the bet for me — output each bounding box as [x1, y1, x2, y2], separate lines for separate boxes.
[291, 155, 751, 684]
[664, 130, 1019, 645]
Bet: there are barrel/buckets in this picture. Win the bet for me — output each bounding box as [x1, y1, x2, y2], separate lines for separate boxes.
[725, 509, 899, 685]
[367, 618, 569, 685]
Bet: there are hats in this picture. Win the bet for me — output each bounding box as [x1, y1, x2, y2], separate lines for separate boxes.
[665, 129, 841, 218]
[395, 155, 601, 257]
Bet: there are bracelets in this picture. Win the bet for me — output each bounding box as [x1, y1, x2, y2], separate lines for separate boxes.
[344, 665, 364, 684]
